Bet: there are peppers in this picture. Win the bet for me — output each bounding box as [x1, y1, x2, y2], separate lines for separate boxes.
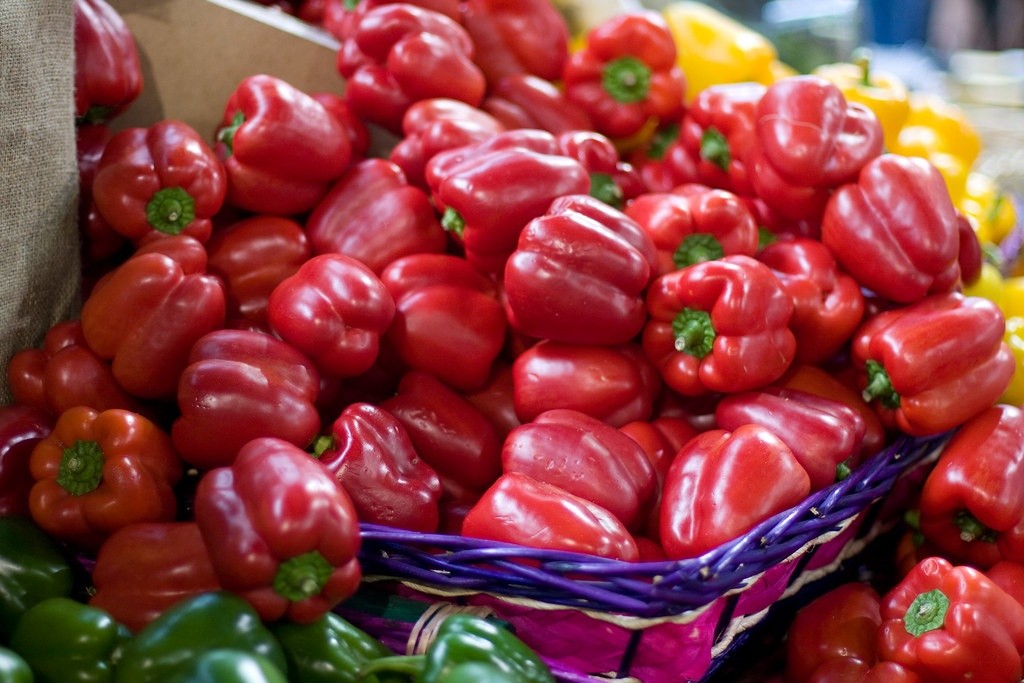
[0, 0, 1024, 683]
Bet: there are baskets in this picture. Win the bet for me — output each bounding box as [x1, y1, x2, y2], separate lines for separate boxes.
[61, 428, 957, 683]
